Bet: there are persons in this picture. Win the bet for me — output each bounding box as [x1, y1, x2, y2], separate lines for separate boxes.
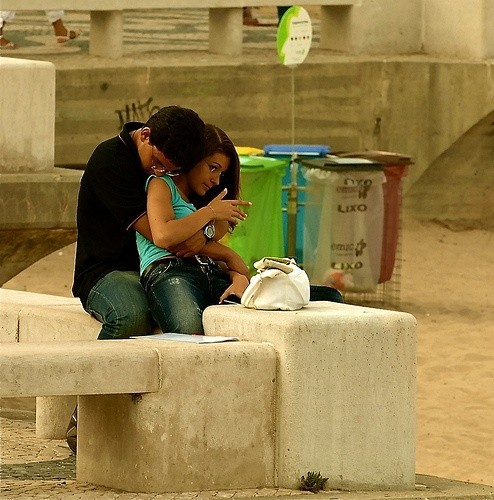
[67, 105, 207, 458]
[137, 123, 254, 339]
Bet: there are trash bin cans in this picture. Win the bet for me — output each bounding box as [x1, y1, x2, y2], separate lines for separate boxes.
[234, 146, 265, 157]
[265, 145, 331, 264]
[295, 157, 386, 293]
[337, 146, 415, 284]
[225, 154, 286, 276]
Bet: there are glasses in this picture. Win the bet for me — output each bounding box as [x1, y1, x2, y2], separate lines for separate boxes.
[150, 144, 180, 178]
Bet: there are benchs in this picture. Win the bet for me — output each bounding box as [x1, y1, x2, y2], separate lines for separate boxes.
[0, 287, 202, 440]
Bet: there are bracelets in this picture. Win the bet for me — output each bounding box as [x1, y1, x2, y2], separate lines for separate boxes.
[208, 205, 216, 223]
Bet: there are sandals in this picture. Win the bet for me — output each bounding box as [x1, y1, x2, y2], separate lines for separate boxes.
[0, 35, 15, 48]
[55, 28, 80, 43]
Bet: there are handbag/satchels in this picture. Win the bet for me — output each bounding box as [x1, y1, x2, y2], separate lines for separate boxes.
[240, 256, 310, 311]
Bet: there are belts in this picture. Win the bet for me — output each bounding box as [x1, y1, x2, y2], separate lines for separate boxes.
[140, 255, 219, 290]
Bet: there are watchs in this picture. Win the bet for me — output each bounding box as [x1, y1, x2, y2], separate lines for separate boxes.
[202, 224, 215, 242]
